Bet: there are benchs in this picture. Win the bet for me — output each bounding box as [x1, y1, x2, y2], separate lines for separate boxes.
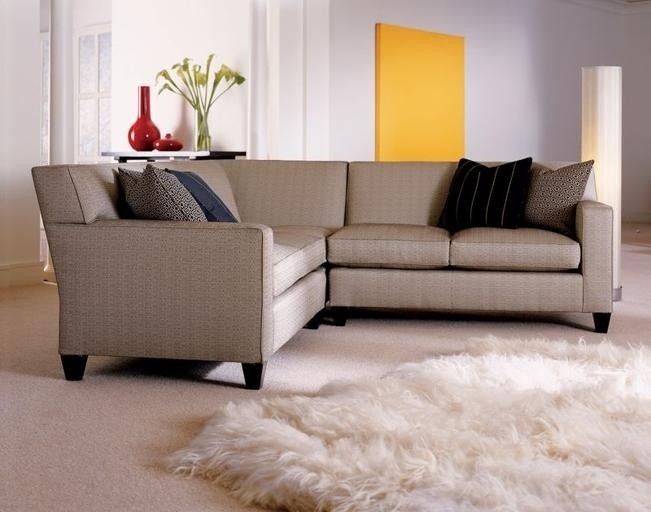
[329, 163, 615, 334]
[28, 163, 346, 391]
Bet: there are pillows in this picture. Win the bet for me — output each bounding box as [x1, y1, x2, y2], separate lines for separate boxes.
[437, 158, 533, 226]
[116, 165, 206, 221]
[526, 159, 595, 233]
[164, 168, 237, 221]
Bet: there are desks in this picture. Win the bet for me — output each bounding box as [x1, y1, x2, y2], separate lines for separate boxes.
[101, 150, 246, 163]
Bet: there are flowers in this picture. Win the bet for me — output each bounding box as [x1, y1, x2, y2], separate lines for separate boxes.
[156, 54, 247, 109]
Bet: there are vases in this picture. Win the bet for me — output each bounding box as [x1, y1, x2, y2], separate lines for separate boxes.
[194, 106, 210, 152]
[128, 85, 160, 151]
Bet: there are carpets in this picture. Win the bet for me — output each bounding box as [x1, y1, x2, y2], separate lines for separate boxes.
[165, 338, 651, 512]
